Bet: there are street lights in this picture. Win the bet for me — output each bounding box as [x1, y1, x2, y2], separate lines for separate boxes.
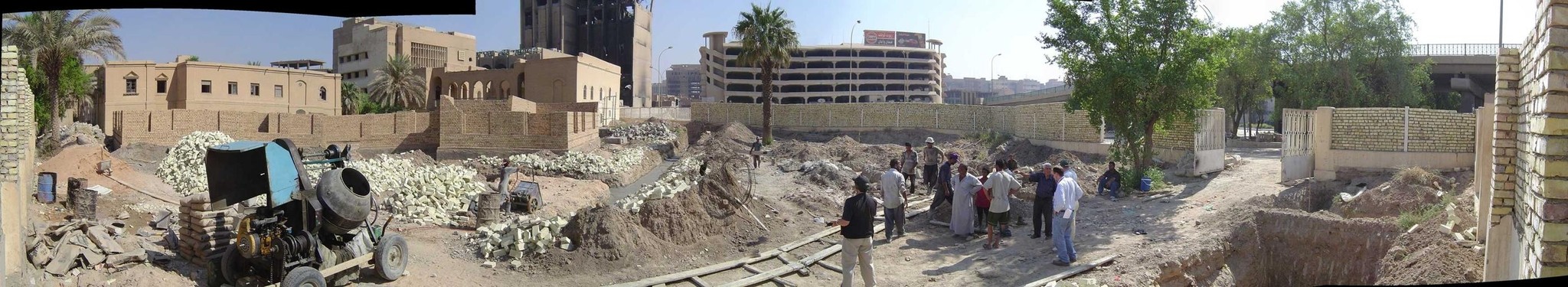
[650, 66, 673, 107]
[991, 54, 1001, 101]
[849, 20, 861, 103]
[658, 46, 673, 123]
[998, 75, 1005, 101]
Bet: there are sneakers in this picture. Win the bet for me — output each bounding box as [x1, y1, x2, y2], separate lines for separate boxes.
[898, 232, 909, 236]
[966, 236, 974, 241]
[1053, 259, 1070, 266]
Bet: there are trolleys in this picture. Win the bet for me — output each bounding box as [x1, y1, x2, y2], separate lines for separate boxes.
[509, 161, 544, 214]
[94, 160, 113, 176]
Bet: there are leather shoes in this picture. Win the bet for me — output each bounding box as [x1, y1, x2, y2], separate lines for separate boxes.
[1031, 234, 1041, 238]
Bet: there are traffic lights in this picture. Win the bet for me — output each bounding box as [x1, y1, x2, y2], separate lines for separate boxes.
[676, 98, 678, 106]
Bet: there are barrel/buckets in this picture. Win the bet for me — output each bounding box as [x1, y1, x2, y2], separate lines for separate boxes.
[477, 192, 503, 225]
[1140, 177, 1152, 191]
[317, 168, 372, 235]
[39, 171, 56, 203]
[68, 178, 88, 205]
[73, 188, 97, 220]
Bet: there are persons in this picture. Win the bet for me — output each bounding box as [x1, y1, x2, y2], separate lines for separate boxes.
[829, 174, 877, 287]
[748, 135, 763, 168]
[922, 137, 944, 194]
[497, 157, 529, 216]
[950, 156, 1083, 268]
[880, 158, 908, 243]
[900, 142, 918, 195]
[929, 154, 958, 212]
[1095, 161, 1121, 202]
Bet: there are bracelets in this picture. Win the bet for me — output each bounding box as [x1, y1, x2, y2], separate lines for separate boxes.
[835, 222, 839, 225]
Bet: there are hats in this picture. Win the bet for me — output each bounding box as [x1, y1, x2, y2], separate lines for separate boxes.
[925, 137, 934, 143]
[853, 175, 870, 188]
[949, 153, 960, 162]
[1060, 160, 1069, 167]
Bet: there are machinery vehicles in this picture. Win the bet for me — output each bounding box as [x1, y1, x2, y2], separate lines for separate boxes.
[203, 137, 408, 287]
